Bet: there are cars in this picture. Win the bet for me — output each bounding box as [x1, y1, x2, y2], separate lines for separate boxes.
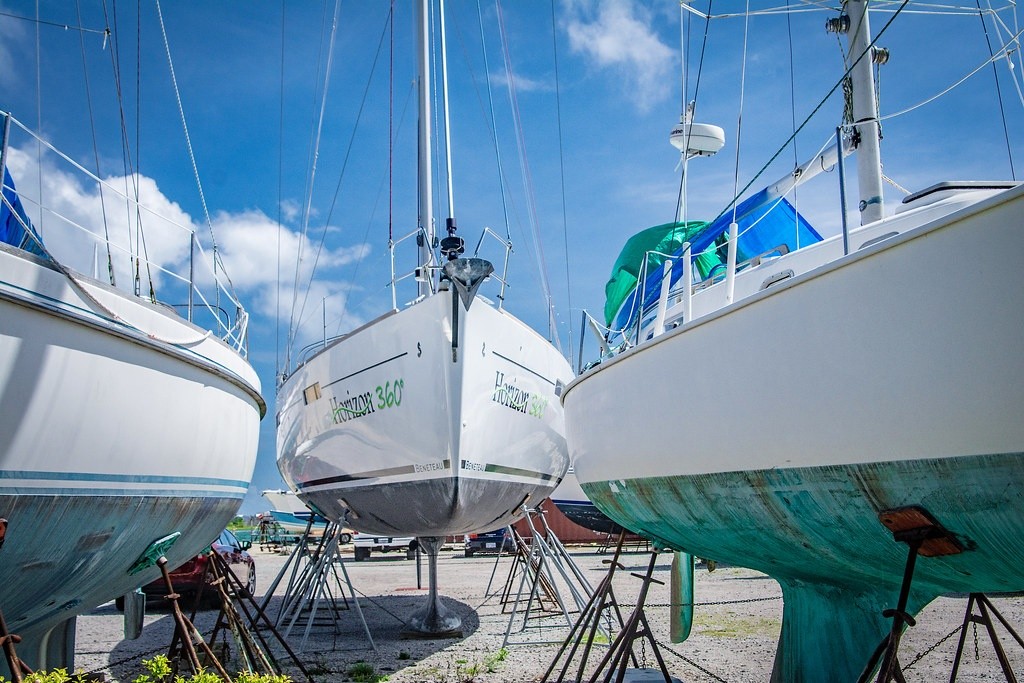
[353, 531, 419, 562]
[113, 528, 257, 612]
[463, 525, 520, 558]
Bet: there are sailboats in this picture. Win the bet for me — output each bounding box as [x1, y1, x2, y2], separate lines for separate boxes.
[556, 1, 1024, 600]
[272, 2, 580, 543]
[0, 1, 264, 650]
